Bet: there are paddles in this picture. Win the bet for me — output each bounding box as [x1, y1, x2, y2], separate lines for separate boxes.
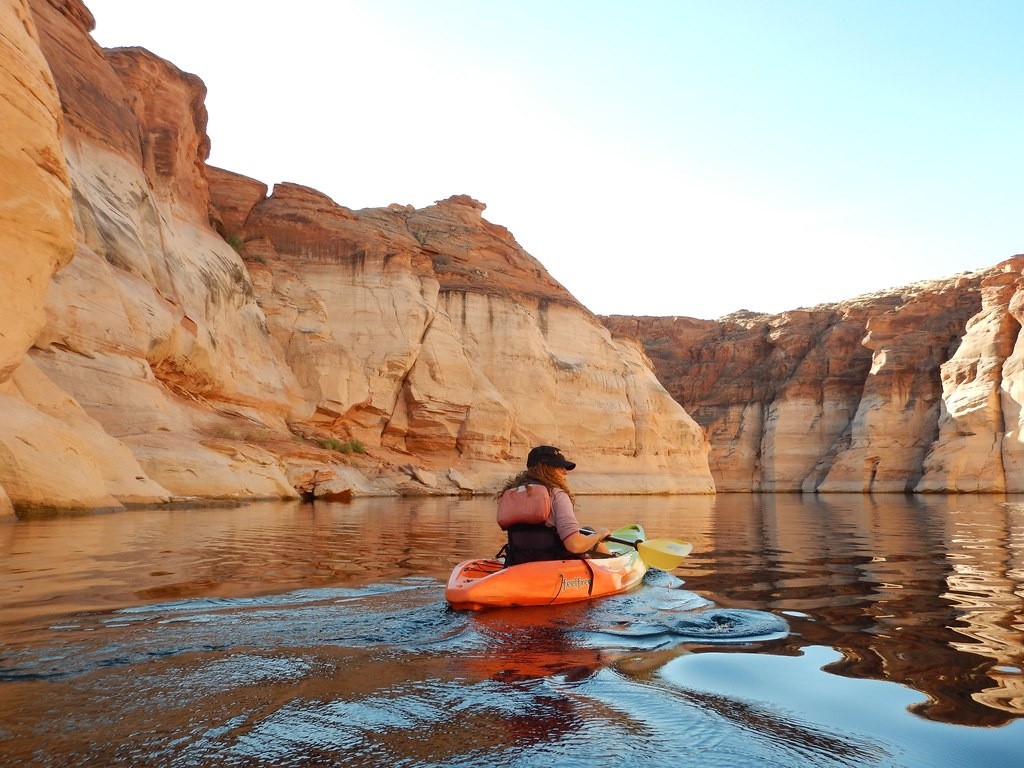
[579, 528, 692, 572]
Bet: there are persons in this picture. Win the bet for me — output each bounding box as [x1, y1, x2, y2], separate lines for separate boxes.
[508, 446, 622, 565]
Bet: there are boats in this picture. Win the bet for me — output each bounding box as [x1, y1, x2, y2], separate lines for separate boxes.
[445, 523, 650, 610]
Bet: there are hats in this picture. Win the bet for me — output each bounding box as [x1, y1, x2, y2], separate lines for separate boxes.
[526, 445, 577, 471]
